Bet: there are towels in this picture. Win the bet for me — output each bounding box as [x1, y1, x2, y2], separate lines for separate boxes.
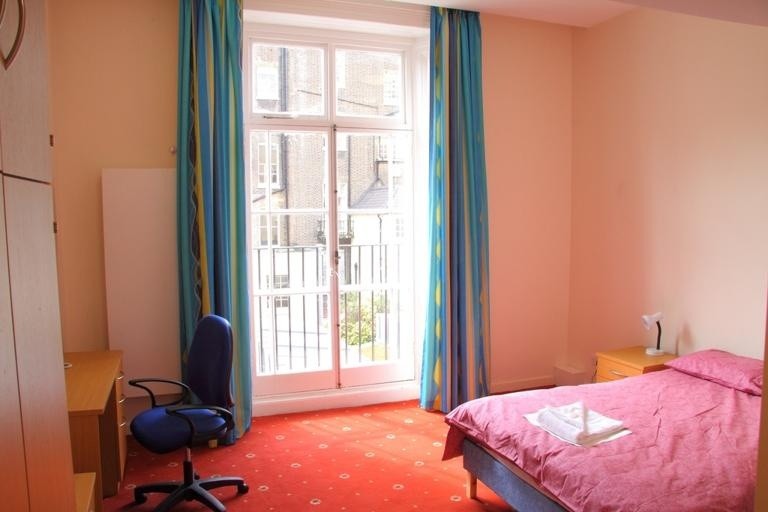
[537, 401, 624, 445]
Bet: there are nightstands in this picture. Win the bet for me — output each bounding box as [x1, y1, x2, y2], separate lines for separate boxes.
[593, 344, 678, 382]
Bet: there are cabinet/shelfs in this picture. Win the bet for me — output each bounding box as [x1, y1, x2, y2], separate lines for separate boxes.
[60, 348, 131, 512]
[1, 0, 81, 512]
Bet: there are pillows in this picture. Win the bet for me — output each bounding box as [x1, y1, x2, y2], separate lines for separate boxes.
[663, 347, 764, 399]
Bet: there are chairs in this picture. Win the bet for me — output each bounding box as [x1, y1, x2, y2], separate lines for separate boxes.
[126, 316, 249, 512]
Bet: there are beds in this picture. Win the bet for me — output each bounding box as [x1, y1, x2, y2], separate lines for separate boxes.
[441, 349, 762, 512]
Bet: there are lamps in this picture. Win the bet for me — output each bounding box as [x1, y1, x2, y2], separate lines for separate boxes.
[641, 311, 666, 356]
